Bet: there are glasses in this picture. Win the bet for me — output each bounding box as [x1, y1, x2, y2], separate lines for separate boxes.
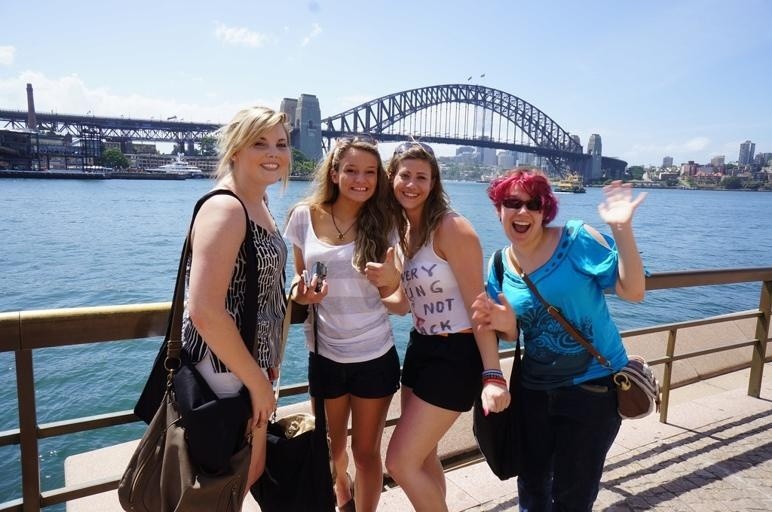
[502, 195, 544, 211]
[314, 262, 327, 291]
[392, 141, 435, 161]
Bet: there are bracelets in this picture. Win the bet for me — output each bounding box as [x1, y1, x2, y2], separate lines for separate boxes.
[481, 367, 508, 386]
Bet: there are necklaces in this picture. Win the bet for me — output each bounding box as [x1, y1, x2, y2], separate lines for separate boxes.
[330, 199, 360, 241]
[515, 250, 529, 278]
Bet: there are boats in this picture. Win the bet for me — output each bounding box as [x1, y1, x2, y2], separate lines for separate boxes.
[143, 151, 204, 175]
[554, 170, 588, 194]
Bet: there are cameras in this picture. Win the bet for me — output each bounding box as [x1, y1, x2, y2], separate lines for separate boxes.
[301, 261, 327, 292]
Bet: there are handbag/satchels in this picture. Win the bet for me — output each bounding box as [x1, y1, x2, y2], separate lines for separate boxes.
[614, 354, 659, 419]
[474, 396, 524, 480]
[118, 340, 336, 512]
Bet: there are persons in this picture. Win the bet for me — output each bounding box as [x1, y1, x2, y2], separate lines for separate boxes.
[181, 103, 294, 512]
[376, 138, 513, 511]
[467, 162, 649, 512]
[281, 135, 403, 511]
